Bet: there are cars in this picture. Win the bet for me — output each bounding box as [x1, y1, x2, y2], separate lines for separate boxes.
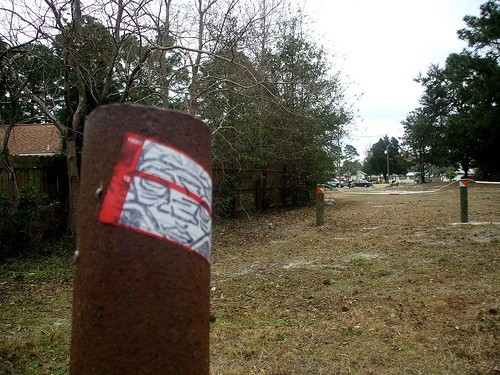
[348, 180, 373, 187]
[316, 177, 343, 188]
[337, 178, 349, 187]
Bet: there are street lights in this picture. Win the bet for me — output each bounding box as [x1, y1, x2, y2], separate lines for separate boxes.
[386, 146, 390, 184]
